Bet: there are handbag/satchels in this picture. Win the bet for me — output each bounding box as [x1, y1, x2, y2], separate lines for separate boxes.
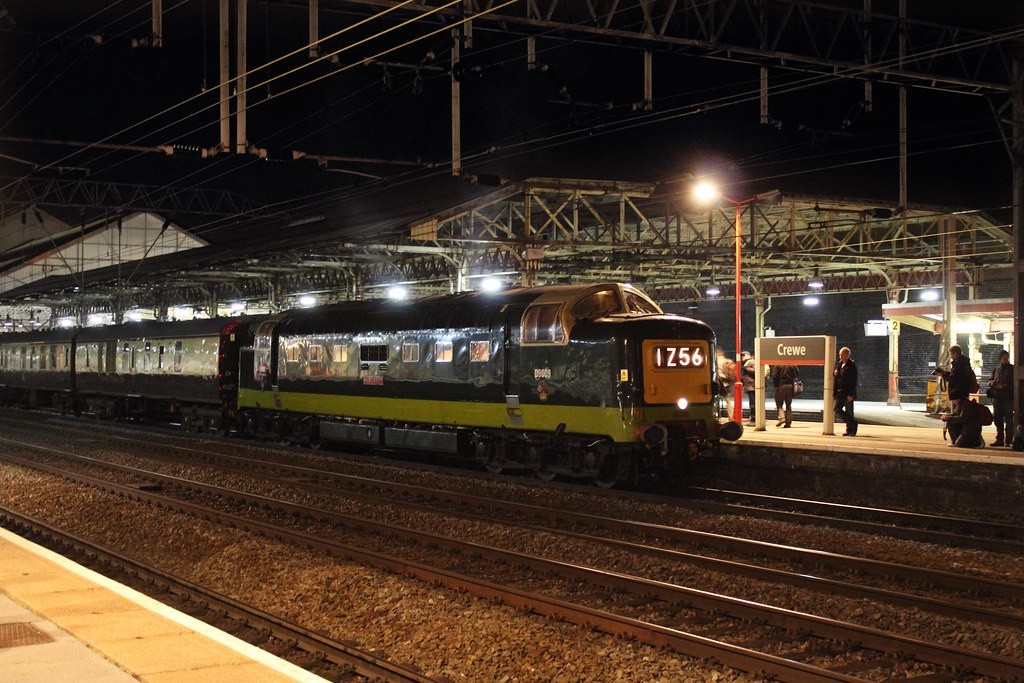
[794, 381, 803, 395]
[986, 389, 994, 398]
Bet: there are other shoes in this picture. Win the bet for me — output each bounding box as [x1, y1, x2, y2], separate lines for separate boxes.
[776, 417, 786, 427]
[948, 443, 957, 447]
[1005, 443, 1012, 447]
[748, 422, 755, 426]
[991, 440, 1004, 446]
[784, 424, 790, 427]
[843, 420, 857, 436]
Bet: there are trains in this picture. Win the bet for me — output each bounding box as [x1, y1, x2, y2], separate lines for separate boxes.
[0, 282, 747, 495]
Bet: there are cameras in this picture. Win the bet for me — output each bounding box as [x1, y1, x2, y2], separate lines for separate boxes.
[931, 366, 944, 375]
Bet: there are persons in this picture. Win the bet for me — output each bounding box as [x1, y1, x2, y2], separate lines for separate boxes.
[715, 345, 734, 422]
[942, 346, 971, 415]
[740, 351, 755, 427]
[832, 346, 858, 436]
[940, 389, 985, 449]
[771, 365, 799, 428]
[987, 350, 1014, 448]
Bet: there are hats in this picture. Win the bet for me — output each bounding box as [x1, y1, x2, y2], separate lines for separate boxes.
[949, 389, 962, 400]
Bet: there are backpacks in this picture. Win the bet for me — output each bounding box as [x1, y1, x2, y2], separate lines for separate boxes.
[723, 362, 737, 381]
[969, 370, 979, 393]
[969, 399, 993, 425]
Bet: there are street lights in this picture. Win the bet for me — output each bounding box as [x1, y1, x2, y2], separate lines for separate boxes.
[695, 180, 781, 426]
